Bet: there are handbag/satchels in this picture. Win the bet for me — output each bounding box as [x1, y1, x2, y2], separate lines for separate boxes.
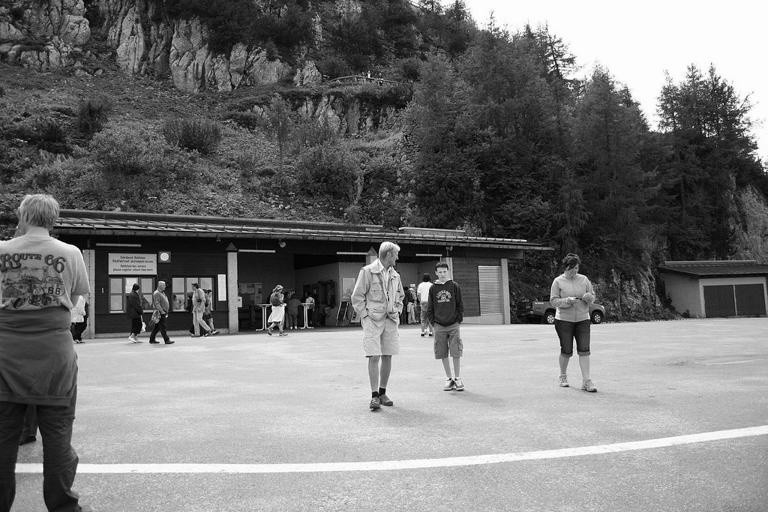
[151, 309, 160, 323]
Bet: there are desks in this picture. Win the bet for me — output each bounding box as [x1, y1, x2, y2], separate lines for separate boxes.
[255, 302, 274, 334]
[298, 302, 315, 331]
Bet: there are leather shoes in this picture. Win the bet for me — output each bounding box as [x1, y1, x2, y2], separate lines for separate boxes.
[149, 339, 159, 343]
[166, 340, 174, 343]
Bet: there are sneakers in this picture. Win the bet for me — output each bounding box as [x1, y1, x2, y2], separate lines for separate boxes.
[370, 395, 393, 409]
[559, 375, 568, 386]
[582, 381, 597, 392]
[444, 378, 464, 390]
[129, 335, 137, 343]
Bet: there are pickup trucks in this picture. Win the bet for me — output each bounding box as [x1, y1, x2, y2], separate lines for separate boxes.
[529, 297, 607, 324]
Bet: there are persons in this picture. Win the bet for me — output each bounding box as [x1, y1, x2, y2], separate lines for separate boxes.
[126, 283, 144, 343]
[173, 293, 193, 312]
[188, 283, 220, 337]
[0, 193, 90, 511]
[17, 405, 38, 445]
[417, 275, 433, 336]
[265, 284, 320, 336]
[70, 294, 88, 344]
[550, 252, 598, 392]
[403, 286, 421, 324]
[350, 241, 405, 411]
[149, 281, 175, 345]
[426, 262, 465, 390]
